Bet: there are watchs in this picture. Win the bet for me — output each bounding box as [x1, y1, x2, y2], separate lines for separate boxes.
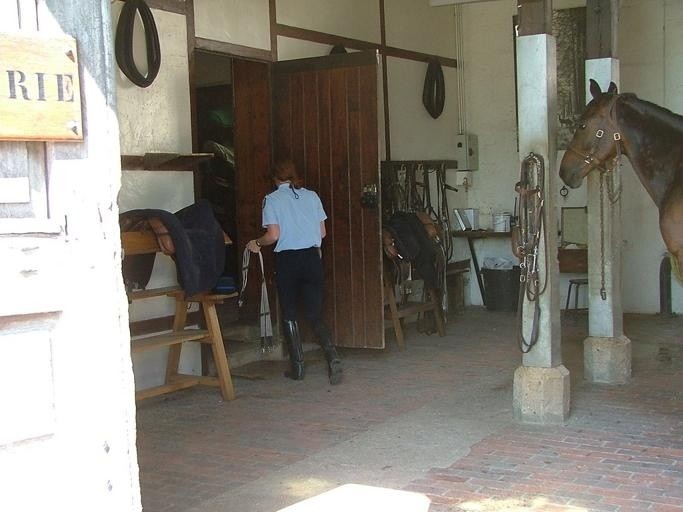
[255, 238, 264, 248]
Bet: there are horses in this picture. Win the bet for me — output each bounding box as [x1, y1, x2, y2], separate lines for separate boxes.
[559, 79, 683, 281]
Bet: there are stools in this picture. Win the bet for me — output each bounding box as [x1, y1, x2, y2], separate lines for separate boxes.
[565, 279, 588, 310]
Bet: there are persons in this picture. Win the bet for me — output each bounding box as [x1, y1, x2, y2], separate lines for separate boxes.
[245, 159, 343, 386]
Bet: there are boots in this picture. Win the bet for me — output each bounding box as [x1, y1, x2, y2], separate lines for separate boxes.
[316, 325, 342, 384]
[283, 320, 305, 379]
